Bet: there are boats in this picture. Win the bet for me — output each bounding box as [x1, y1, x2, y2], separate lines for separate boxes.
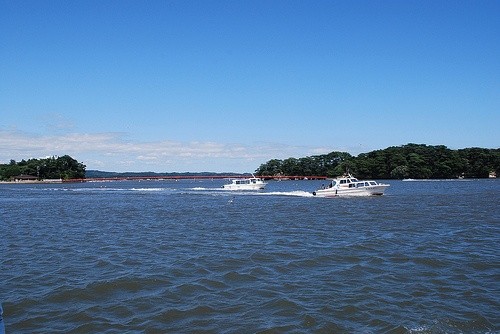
[220, 177, 269, 189]
[312, 173, 391, 196]
[489, 171, 497, 178]
